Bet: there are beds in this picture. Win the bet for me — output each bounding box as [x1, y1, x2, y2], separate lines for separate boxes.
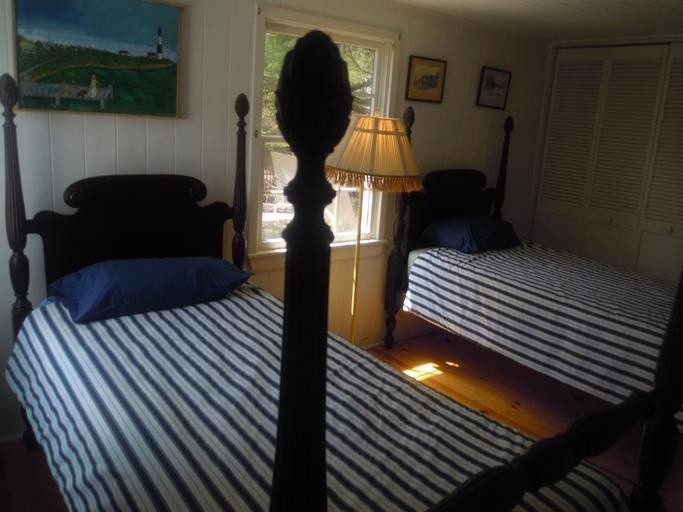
[382, 106, 683, 433]
[0, 71, 683, 510]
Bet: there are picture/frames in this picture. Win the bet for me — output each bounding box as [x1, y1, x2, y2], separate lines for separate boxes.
[10, 0, 186, 123]
[474, 65, 513, 110]
[403, 54, 448, 105]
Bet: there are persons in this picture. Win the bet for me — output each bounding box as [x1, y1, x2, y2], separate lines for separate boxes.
[87, 73, 102, 98]
[69, 80, 87, 98]
[487, 75, 496, 96]
[46, 81, 67, 96]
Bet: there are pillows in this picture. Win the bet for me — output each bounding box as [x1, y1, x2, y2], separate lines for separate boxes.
[46, 254, 253, 326]
[422, 215, 521, 254]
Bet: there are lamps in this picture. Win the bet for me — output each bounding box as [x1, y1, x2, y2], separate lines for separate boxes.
[322, 114, 423, 346]
